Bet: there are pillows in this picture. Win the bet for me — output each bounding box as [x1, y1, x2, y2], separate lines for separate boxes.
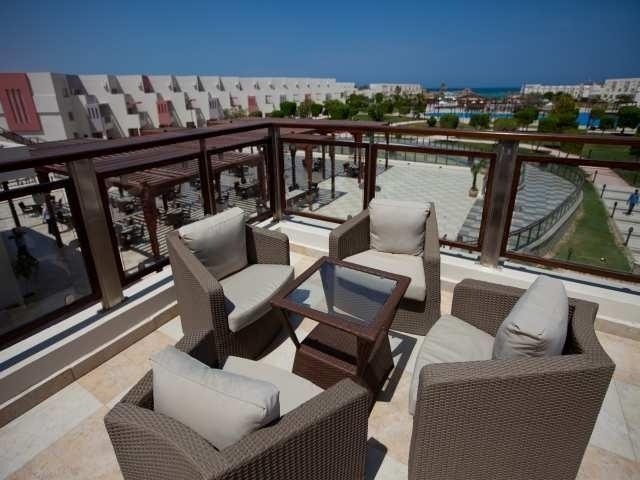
[492, 275, 571, 358]
[367, 198, 432, 255]
[148, 345, 280, 452]
[178, 208, 248, 281]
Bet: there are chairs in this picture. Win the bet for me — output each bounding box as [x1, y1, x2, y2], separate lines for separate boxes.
[14, 176, 197, 252]
[206, 142, 361, 213]
[409, 278, 616, 480]
[329, 198, 441, 336]
[165, 208, 294, 366]
[104, 329, 369, 479]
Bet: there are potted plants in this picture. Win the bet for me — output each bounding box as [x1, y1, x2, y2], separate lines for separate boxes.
[468, 159, 482, 197]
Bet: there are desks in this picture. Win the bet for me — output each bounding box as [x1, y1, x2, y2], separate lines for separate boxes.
[271, 256, 410, 399]
